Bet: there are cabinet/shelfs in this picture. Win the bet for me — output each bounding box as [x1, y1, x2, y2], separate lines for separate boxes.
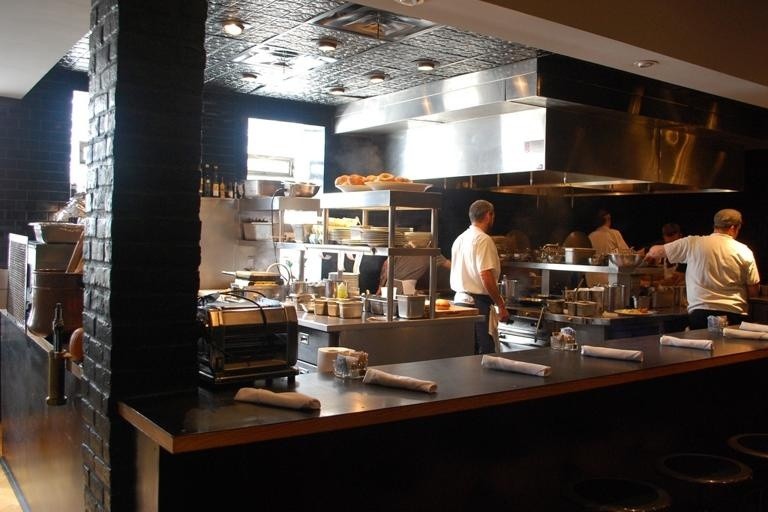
[233, 192, 441, 323]
[493, 258, 690, 311]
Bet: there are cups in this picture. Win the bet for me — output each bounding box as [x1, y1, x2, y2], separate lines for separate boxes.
[548, 299, 566, 314]
[324, 279, 347, 298]
[631, 295, 653, 309]
[402, 279, 418, 296]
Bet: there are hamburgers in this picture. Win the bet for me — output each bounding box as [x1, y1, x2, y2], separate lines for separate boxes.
[436, 299, 450, 310]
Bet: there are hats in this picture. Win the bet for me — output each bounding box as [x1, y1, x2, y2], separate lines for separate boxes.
[714, 209, 741, 227]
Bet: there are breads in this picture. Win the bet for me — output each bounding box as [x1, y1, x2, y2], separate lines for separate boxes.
[335, 173, 411, 189]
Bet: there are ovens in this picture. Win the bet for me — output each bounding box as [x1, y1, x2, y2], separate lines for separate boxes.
[497, 328, 549, 355]
[283, 326, 337, 376]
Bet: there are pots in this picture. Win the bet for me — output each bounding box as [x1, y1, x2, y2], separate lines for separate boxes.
[497, 274, 520, 296]
[516, 297, 542, 307]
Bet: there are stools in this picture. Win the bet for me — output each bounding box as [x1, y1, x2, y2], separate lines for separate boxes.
[727, 422, 767, 511]
[664, 451, 751, 512]
[561, 474, 673, 511]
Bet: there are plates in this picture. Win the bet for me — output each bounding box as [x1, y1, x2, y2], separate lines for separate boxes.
[335, 184, 369, 192]
[614, 309, 658, 315]
[365, 181, 433, 191]
[327, 272, 360, 296]
[330, 226, 417, 247]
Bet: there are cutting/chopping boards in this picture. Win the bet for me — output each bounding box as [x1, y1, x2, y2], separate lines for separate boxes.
[435, 305, 479, 318]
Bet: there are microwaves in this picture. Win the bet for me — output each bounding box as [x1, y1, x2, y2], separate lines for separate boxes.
[198, 296, 302, 386]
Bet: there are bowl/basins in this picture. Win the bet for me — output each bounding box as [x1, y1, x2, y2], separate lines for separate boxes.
[587, 258, 598, 266]
[565, 247, 596, 263]
[405, 232, 434, 248]
[607, 253, 645, 267]
[313, 299, 364, 319]
[292, 185, 321, 198]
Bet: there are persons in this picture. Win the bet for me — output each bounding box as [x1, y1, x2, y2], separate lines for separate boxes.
[646, 207, 762, 332]
[655, 221, 687, 273]
[374, 245, 451, 296]
[582, 208, 631, 289]
[449, 198, 510, 355]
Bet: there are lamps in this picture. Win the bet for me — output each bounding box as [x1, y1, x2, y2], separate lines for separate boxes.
[223, 19, 443, 98]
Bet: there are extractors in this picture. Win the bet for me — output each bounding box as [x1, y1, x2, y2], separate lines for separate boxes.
[384, 105, 748, 197]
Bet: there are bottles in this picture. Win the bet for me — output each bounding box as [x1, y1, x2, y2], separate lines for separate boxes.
[333, 356, 367, 379]
[199, 160, 246, 200]
[707, 314, 729, 333]
[550, 331, 579, 350]
